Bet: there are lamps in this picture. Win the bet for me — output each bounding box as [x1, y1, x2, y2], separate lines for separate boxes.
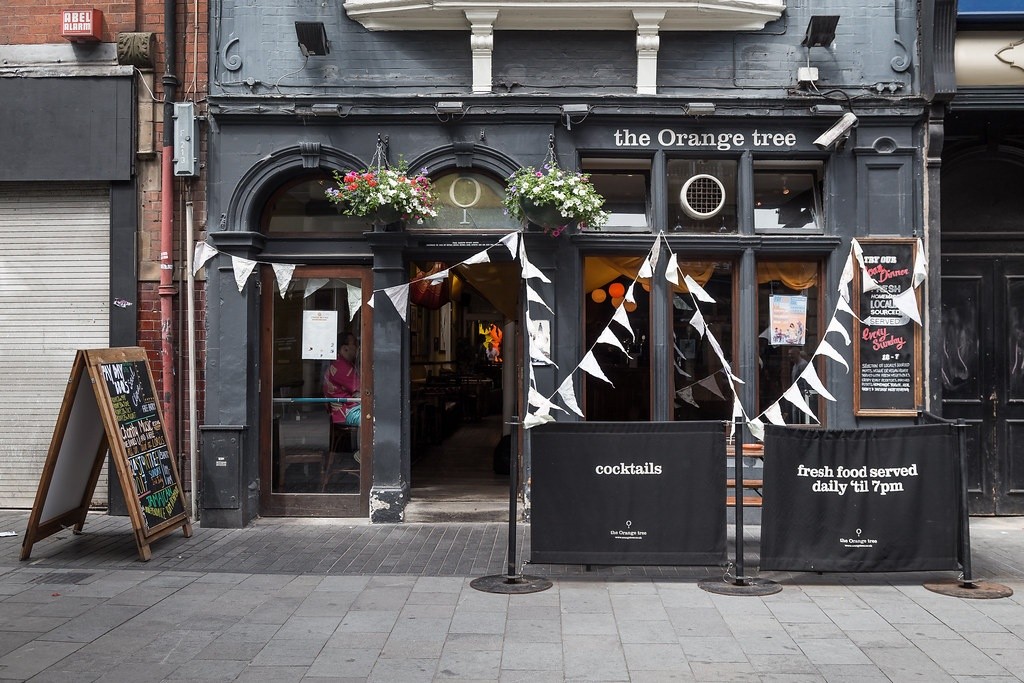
[800, 14, 840, 48]
[797, 66, 819, 86]
[810, 104, 843, 116]
[685, 103, 715, 116]
[591, 283, 637, 312]
[311, 103, 342, 116]
[295, 20, 332, 56]
[562, 104, 590, 116]
[435, 101, 465, 114]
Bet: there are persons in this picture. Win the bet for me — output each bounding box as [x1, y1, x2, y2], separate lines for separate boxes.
[456, 334, 503, 367]
[788, 347, 812, 424]
[322, 332, 360, 465]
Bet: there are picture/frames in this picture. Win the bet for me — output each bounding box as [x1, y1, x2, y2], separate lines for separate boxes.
[678, 337, 697, 360]
[851, 236, 923, 417]
[410, 304, 418, 332]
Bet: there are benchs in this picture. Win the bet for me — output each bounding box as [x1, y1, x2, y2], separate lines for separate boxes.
[724, 444, 764, 507]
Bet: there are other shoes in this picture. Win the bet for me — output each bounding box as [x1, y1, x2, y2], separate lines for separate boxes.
[353, 450, 360, 463]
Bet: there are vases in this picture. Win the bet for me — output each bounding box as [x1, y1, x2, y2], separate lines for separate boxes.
[520, 195, 587, 229]
[342, 198, 409, 222]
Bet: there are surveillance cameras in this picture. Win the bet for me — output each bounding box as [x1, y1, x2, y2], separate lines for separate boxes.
[813, 113, 857, 151]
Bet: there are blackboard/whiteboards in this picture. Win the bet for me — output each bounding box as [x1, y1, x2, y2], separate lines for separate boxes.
[15, 345, 189, 546]
[852, 236, 925, 418]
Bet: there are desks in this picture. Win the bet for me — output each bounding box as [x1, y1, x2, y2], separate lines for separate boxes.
[412, 378, 494, 411]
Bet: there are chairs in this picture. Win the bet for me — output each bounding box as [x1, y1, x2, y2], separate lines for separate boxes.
[277, 372, 478, 492]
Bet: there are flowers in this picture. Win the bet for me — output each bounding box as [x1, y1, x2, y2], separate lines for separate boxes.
[316, 153, 440, 224]
[500, 164, 611, 236]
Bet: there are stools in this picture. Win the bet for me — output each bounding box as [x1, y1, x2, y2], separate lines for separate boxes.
[321, 426, 360, 493]
